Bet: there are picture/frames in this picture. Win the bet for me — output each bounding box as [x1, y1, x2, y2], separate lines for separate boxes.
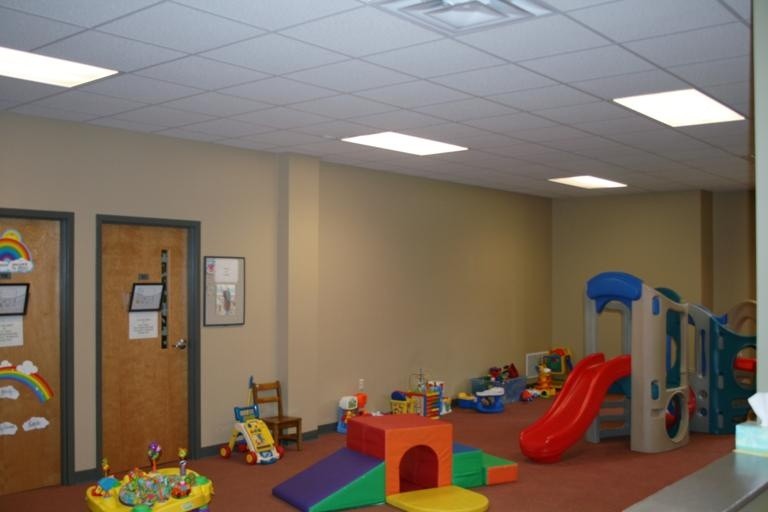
[128, 283, 165, 312]
[0, 283, 31, 317]
[203, 255, 245, 330]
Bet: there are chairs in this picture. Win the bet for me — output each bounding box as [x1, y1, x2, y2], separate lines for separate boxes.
[248, 382, 303, 454]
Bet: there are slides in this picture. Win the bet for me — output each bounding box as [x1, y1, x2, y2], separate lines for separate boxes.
[519, 353, 631, 463]
